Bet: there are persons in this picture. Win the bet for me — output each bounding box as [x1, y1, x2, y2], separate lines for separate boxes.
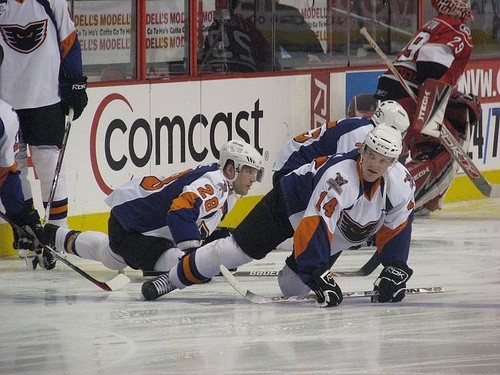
[271, 100, 410, 188]
[0, 0, 88, 260]
[200, 0, 324, 75]
[374, 0, 480, 217]
[36, 141, 265, 279]
[141, 122, 416, 308]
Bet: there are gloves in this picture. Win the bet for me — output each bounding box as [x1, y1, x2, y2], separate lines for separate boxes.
[59, 75, 88, 122]
[370, 263, 413, 303]
[307, 267, 343, 307]
[4, 198, 43, 253]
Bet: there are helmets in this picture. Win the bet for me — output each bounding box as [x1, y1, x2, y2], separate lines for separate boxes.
[370, 100, 410, 136]
[358, 123, 403, 169]
[218, 139, 264, 176]
[432, 0, 472, 20]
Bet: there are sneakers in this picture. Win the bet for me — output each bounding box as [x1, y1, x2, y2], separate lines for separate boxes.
[137, 272, 176, 301]
[38, 222, 59, 270]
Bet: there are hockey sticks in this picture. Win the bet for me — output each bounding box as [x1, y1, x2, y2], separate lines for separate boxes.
[219, 263, 446, 303]
[24, 108, 75, 272]
[0, 210, 131, 292]
[118, 247, 380, 277]
[359, 26, 499, 198]
